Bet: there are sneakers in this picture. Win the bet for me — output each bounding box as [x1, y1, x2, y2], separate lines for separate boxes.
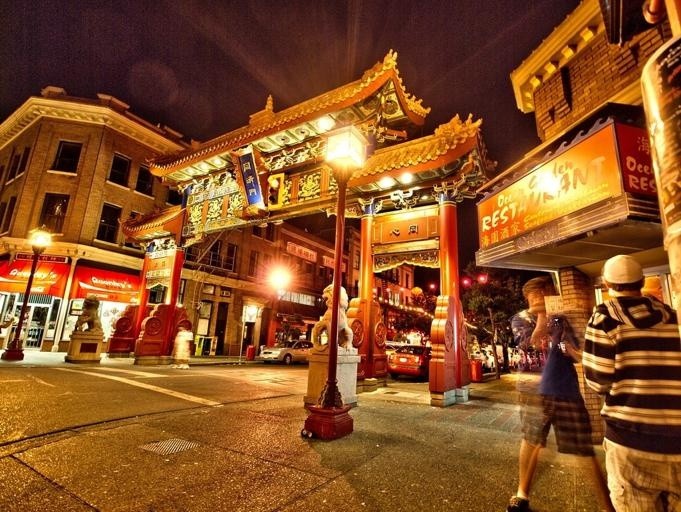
[505, 495, 530, 512]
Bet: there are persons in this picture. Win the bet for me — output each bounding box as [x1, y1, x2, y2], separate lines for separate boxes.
[168, 324, 192, 370]
[507, 276, 616, 511]
[582, 254, 681, 512]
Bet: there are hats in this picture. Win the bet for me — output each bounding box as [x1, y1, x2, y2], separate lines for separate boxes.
[602, 254, 645, 286]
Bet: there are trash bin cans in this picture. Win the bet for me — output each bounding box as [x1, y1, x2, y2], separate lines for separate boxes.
[246, 345, 257, 361]
[470, 359, 483, 384]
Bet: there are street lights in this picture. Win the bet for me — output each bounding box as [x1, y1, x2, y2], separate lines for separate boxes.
[0, 222, 53, 361]
[296, 117, 375, 442]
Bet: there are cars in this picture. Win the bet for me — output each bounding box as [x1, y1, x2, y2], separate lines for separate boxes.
[257, 339, 313, 366]
[380, 338, 545, 381]
